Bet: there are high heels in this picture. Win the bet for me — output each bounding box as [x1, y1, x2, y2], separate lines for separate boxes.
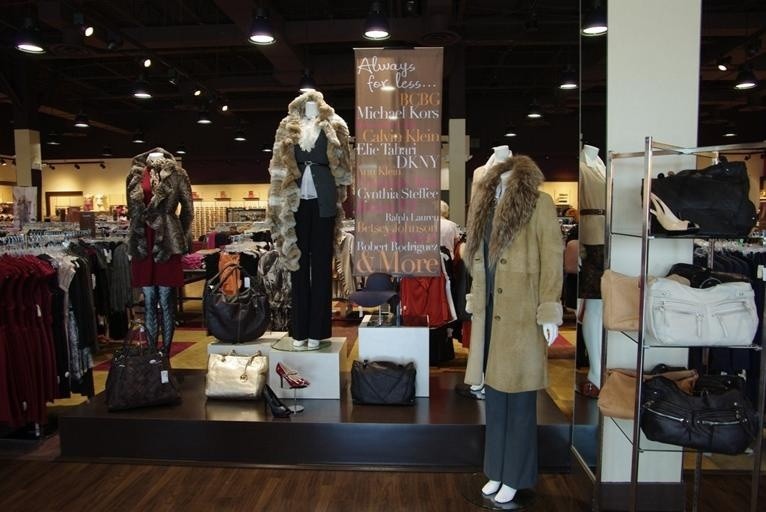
[260, 384, 291, 418]
[276, 362, 310, 388]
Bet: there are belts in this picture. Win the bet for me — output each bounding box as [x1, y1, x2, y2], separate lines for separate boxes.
[581, 209, 606, 215]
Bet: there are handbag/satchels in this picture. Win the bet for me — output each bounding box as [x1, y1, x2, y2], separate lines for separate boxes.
[600, 270, 690, 331]
[639, 376, 757, 455]
[640, 161, 757, 238]
[205, 349, 270, 399]
[203, 264, 271, 342]
[351, 359, 417, 405]
[106, 323, 182, 410]
[597, 369, 699, 419]
[646, 278, 758, 347]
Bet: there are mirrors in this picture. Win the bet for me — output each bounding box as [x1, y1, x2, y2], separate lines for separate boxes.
[569, 1, 609, 476]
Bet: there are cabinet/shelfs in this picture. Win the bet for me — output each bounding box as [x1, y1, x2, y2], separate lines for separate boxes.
[598, 142, 766, 510]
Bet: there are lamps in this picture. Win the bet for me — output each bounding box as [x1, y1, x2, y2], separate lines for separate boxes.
[361, 8, 391, 43]
[581, 14, 607, 37]
[248, 10, 275, 49]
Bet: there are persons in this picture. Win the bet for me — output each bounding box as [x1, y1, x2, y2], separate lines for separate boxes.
[268, 88, 354, 350]
[123, 151, 195, 360]
[579, 144, 608, 398]
[470, 145, 514, 394]
[462, 168, 564, 504]
[439, 197, 461, 267]
